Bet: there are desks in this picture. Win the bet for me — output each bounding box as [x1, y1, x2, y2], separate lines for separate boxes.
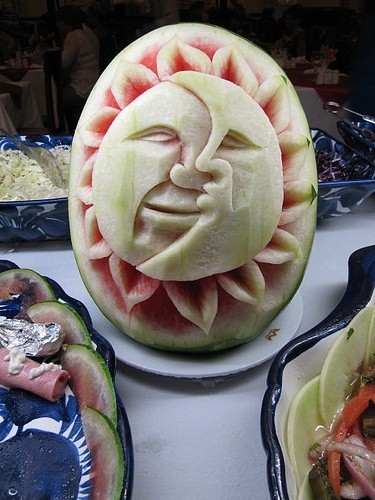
[285, 63, 352, 140]
[0, 81, 45, 139]
[0, 62, 60, 128]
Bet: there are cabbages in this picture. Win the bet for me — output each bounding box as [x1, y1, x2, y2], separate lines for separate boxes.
[0, 142, 70, 202]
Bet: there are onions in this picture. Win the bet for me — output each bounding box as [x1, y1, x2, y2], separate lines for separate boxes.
[306, 432, 375, 500]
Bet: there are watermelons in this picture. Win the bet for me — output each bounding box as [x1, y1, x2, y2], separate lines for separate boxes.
[0, 268, 126, 500]
[65, 23, 319, 355]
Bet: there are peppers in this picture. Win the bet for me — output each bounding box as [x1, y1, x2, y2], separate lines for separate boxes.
[321, 381, 375, 499]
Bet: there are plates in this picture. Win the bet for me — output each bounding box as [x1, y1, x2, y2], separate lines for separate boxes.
[74, 288, 304, 379]
[0, 259, 135, 500]
[261, 246, 375, 500]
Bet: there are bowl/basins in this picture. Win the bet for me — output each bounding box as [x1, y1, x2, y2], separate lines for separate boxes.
[0, 67, 28, 82]
[310, 127, 375, 225]
[338, 120, 375, 165]
[0, 133, 72, 241]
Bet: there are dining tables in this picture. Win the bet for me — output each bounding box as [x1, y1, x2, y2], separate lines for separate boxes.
[0, 195, 375, 500]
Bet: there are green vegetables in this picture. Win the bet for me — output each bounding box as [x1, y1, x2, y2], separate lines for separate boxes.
[311, 328, 375, 447]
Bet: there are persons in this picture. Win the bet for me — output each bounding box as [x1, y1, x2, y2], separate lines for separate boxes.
[0, 0, 374, 134]
